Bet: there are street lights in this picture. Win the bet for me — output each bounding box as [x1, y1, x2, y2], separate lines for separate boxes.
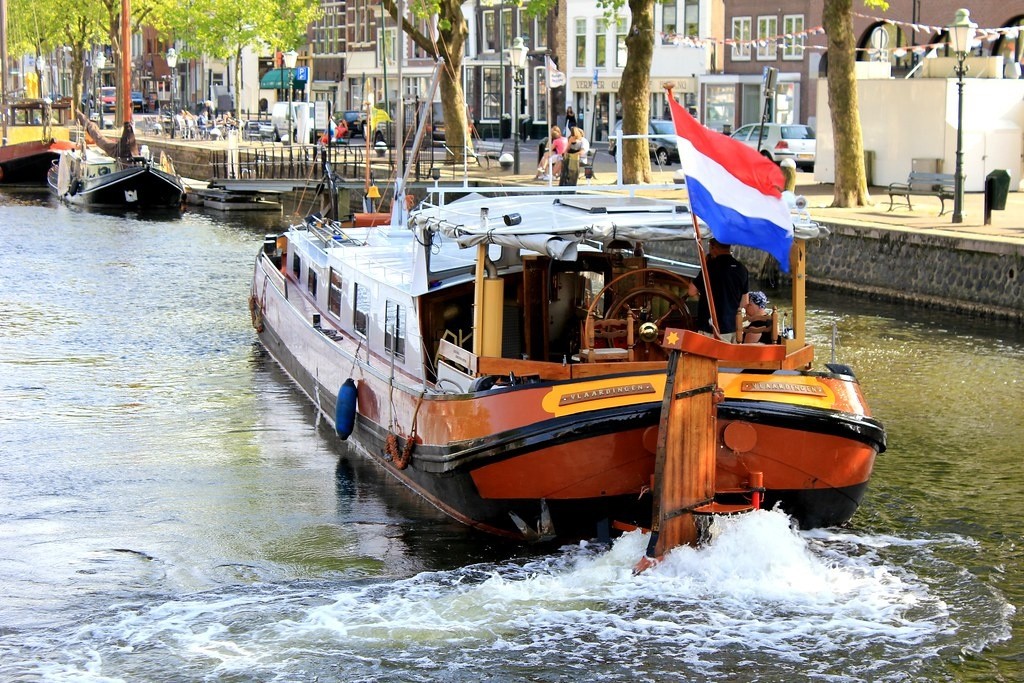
[95, 52, 106, 130]
[166, 48, 178, 139]
[36, 56, 44, 99]
[62, 46, 72, 98]
[508, 37, 529, 175]
[284, 50, 298, 145]
[944, 8, 978, 223]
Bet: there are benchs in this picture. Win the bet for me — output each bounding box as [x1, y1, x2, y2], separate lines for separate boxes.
[883, 171, 967, 218]
[580, 148, 597, 180]
[333, 130, 354, 155]
[458, 140, 504, 171]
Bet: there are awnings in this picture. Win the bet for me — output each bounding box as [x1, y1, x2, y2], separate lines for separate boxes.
[260, 69, 305, 90]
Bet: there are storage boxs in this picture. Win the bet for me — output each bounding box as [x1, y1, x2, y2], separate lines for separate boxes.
[281, 141, 290, 145]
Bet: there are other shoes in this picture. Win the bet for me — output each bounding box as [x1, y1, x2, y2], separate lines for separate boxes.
[534, 167, 558, 181]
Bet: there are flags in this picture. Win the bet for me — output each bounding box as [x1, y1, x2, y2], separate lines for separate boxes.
[668, 92, 794, 274]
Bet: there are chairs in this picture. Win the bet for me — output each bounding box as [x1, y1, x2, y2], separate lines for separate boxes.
[248, 124, 275, 147]
[578, 310, 635, 364]
[736, 305, 780, 345]
[143, 115, 245, 141]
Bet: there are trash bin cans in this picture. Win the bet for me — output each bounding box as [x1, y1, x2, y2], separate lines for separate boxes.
[864, 150, 876, 186]
[984, 169, 1012, 210]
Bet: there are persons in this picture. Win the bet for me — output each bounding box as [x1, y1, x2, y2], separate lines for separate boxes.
[741, 292, 780, 345]
[687, 238, 750, 345]
[564, 106, 577, 130]
[318, 119, 348, 146]
[176, 109, 231, 140]
[534, 125, 589, 182]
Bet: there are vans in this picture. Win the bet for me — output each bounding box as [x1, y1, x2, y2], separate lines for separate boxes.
[271, 102, 337, 144]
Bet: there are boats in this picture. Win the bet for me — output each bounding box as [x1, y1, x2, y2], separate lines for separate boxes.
[248, 195, 886, 577]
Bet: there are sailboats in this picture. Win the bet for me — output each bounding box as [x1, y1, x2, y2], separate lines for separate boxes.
[47, 0, 183, 211]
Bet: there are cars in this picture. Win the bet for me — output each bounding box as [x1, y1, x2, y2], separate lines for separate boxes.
[131, 92, 149, 113]
[373, 100, 473, 149]
[82, 94, 94, 109]
[332, 110, 367, 138]
[608, 119, 681, 166]
[723, 123, 816, 172]
[688, 106, 732, 132]
[595, 102, 608, 127]
[48, 94, 63, 101]
[96, 87, 117, 113]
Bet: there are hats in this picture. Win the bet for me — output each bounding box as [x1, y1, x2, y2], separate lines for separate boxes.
[749, 291, 768, 308]
